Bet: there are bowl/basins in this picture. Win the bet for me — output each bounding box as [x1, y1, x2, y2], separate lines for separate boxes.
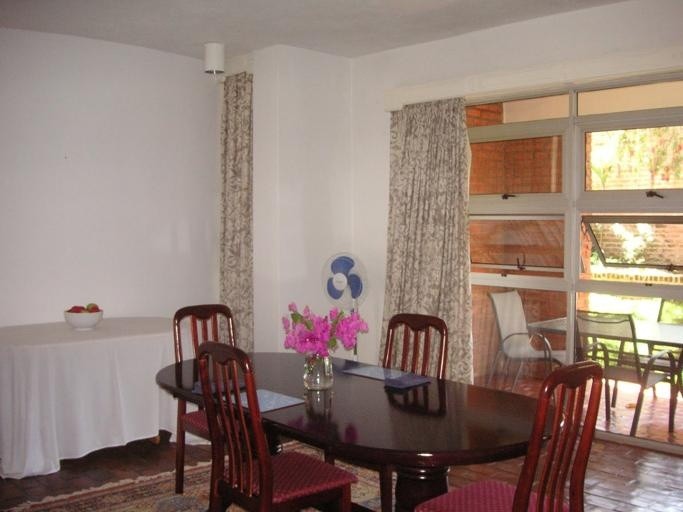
[64, 309, 102, 333]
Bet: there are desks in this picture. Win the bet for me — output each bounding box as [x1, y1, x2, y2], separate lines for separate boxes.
[153, 351, 565, 510]
[0, 315, 180, 479]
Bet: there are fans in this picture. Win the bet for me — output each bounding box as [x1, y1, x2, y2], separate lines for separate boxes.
[321, 252, 371, 362]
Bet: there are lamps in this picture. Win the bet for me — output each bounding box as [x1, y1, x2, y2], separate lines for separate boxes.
[203, 42, 224, 76]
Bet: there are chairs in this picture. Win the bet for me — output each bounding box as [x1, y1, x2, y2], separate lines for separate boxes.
[485, 288, 683, 438]
